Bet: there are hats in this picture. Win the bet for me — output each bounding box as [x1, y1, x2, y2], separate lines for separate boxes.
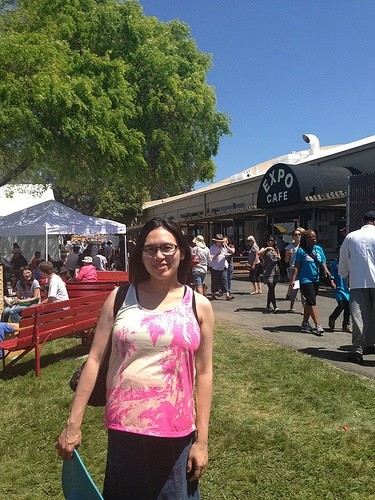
[193, 235, 207, 249]
[12, 248, 21, 254]
[211, 233, 227, 242]
[82, 256, 92, 263]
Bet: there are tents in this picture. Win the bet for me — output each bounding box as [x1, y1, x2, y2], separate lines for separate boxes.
[0, 200, 127, 283]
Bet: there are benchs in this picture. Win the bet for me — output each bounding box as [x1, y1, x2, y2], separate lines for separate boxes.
[96, 271, 129, 286]
[0, 291, 111, 380]
[65, 281, 115, 298]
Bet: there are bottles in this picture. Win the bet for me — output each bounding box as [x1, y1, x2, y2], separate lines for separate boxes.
[5, 282, 13, 296]
[11, 355, 18, 361]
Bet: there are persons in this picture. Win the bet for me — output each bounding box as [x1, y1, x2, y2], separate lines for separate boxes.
[284, 227, 305, 315]
[256, 235, 281, 313]
[338, 211, 375, 363]
[60, 236, 97, 282]
[328, 246, 353, 334]
[0, 243, 70, 306]
[208, 233, 236, 300]
[0, 266, 41, 323]
[0, 323, 15, 358]
[237, 235, 262, 295]
[31, 261, 70, 325]
[79, 238, 135, 272]
[54, 217, 214, 500]
[290, 229, 331, 335]
[185, 235, 212, 297]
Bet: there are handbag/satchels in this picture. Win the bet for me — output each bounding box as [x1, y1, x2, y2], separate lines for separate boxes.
[69, 282, 130, 407]
[191, 247, 200, 264]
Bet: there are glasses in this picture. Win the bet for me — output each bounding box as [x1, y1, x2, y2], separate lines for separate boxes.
[267, 240, 273, 242]
[142, 244, 179, 256]
[294, 233, 301, 236]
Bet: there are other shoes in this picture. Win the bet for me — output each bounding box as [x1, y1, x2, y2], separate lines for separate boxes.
[258, 289, 263, 294]
[301, 321, 314, 333]
[341, 323, 352, 333]
[266, 306, 273, 311]
[328, 315, 337, 330]
[273, 308, 280, 313]
[229, 293, 232, 296]
[354, 352, 365, 363]
[289, 308, 296, 312]
[218, 292, 223, 297]
[250, 291, 258, 294]
[315, 325, 326, 335]
[211, 296, 217, 300]
[226, 295, 235, 300]
